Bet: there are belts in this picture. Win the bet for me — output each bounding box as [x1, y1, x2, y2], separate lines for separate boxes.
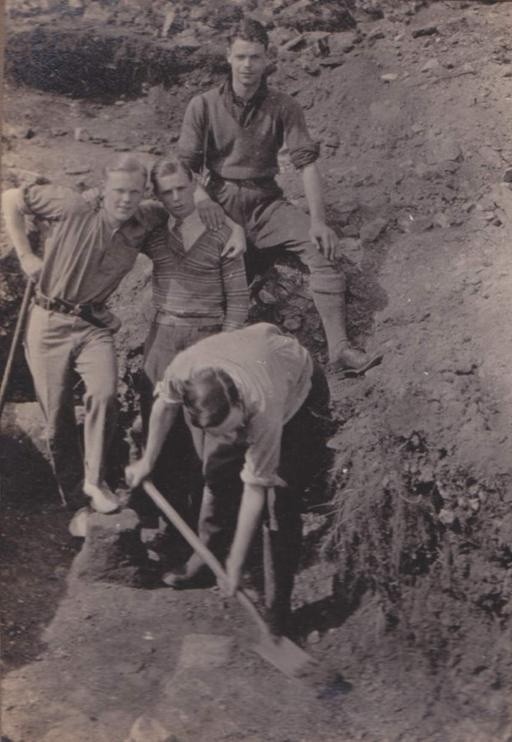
[36, 296, 103, 314]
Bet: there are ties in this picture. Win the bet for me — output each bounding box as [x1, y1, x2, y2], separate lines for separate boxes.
[170, 221, 184, 253]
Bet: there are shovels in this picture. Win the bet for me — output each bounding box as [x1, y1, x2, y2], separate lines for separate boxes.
[141, 477, 319, 678]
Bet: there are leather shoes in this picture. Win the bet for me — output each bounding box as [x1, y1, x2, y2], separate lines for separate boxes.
[326, 344, 384, 376]
[161, 553, 212, 589]
[81, 480, 121, 514]
[68, 506, 91, 538]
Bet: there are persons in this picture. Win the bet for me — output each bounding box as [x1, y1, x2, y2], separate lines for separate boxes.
[175, 16, 383, 381]
[80, 155, 249, 548]
[120, 320, 331, 646]
[1, 155, 249, 539]
